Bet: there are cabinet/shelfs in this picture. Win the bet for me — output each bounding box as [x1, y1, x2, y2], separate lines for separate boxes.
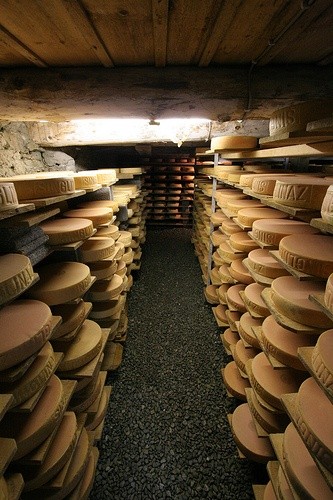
[0, 172, 147, 500]
[190, 141, 333, 500]
[96, 147, 215, 225]
